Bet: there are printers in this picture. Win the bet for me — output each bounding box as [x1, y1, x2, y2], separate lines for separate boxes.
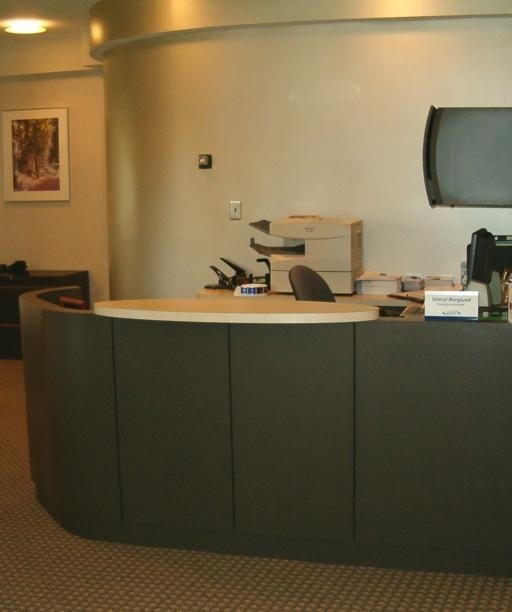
[269, 216, 363, 293]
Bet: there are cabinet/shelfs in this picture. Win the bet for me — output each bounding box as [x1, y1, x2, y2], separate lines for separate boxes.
[0, 269, 89, 360]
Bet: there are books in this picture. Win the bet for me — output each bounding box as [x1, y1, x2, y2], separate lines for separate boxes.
[355, 270, 455, 296]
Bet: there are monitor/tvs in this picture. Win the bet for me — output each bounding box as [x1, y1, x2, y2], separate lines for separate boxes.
[467, 228, 495, 287]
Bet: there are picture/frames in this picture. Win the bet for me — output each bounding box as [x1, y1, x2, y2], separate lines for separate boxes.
[1, 105, 72, 202]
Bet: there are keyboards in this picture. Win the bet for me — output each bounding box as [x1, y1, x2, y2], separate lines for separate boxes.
[400, 303, 424, 317]
